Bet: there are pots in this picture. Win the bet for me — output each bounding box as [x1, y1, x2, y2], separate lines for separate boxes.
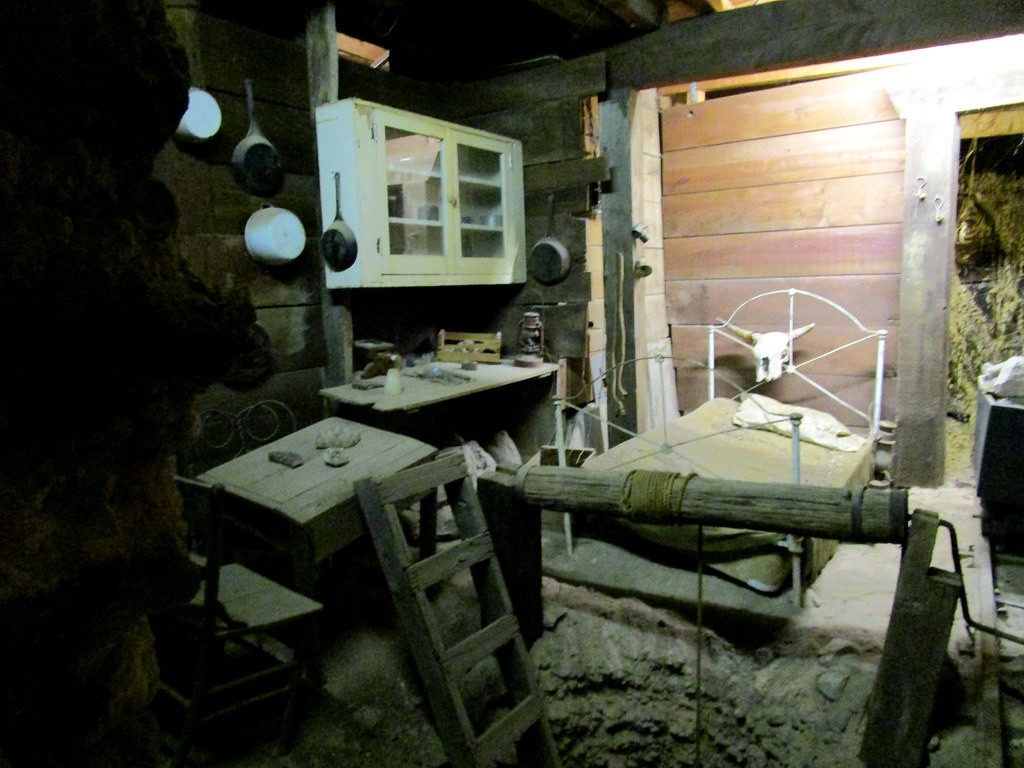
[530, 196, 570, 284]
[320, 172, 355, 273]
[231, 79, 282, 191]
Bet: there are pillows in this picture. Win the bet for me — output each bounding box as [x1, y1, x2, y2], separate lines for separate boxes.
[732, 391, 866, 452]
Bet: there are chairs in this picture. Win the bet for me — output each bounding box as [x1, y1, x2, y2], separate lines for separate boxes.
[148, 471, 326, 768]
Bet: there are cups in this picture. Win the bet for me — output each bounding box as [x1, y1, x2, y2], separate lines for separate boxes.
[480, 215, 502, 226]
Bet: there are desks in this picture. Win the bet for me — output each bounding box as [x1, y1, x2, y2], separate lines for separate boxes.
[195, 416, 440, 658]
[318, 365, 560, 431]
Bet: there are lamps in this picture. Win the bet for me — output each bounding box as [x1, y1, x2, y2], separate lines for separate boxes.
[513, 311, 544, 367]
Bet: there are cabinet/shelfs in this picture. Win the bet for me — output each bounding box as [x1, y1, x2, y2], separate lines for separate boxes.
[314, 96, 528, 290]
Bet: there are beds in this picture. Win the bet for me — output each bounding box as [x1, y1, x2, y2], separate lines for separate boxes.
[476, 285, 963, 768]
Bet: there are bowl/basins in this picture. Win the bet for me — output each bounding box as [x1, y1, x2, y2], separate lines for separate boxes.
[174, 87, 221, 144]
[245, 202, 306, 266]
